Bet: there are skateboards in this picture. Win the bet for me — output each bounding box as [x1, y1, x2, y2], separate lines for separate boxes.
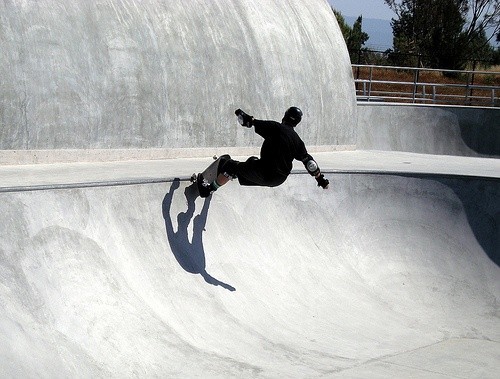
[190, 154, 231, 198]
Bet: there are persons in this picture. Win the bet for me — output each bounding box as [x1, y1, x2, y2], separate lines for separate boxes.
[191, 106, 330, 198]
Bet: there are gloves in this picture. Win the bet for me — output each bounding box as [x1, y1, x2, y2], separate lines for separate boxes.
[235, 109, 253, 128]
[315, 173, 329, 189]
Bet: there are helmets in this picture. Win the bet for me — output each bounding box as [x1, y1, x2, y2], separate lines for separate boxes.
[284, 107, 303, 127]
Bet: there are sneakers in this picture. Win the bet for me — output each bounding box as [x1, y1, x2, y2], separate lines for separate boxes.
[197, 173, 212, 198]
